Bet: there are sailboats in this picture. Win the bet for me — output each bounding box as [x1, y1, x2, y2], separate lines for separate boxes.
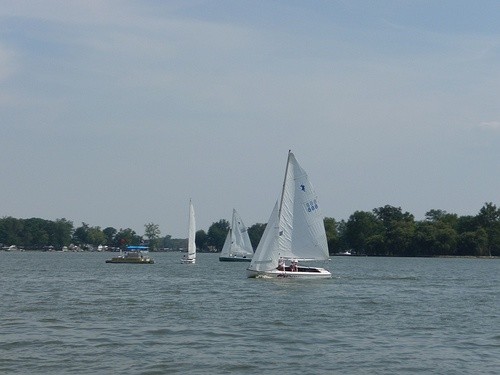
[246, 147, 332, 280]
[180, 197, 197, 264]
[217, 208, 255, 262]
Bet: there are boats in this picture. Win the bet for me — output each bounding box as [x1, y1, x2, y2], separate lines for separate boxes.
[105, 250, 155, 265]
